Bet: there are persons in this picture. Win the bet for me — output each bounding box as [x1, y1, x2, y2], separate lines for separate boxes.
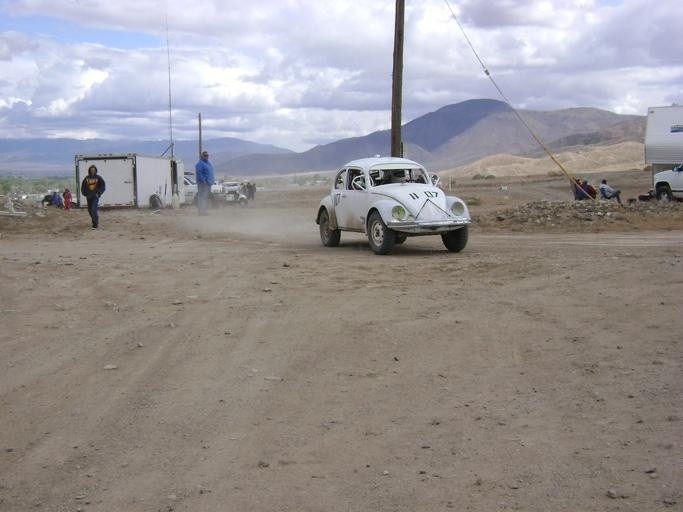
[49, 190, 62, 207]
[382, 170, 407, 185]
[239, 181, 257, 201]
[62, 188, 73, 210]
[194, 151, 216, 216]
[569, 175, 623, 203]
[80, 163, 106, 231]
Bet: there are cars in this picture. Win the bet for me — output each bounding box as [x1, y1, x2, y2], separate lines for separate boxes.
[183, 171, 249, 208]
[313, 151, 471, 252]
